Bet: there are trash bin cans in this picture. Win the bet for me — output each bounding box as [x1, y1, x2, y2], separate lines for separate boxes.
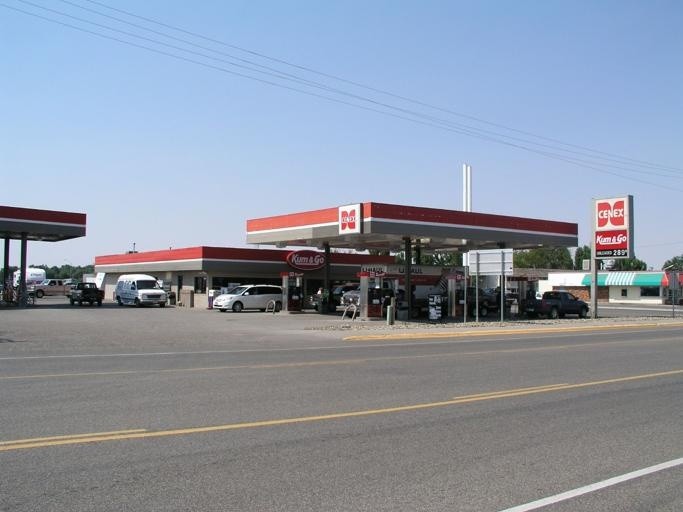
[386, 305, 394, 325]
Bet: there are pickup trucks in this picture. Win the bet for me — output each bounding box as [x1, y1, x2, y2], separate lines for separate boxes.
[68, 282, 105, 307]
[30, 279, 70, 298]
[521, 291, 588, 318]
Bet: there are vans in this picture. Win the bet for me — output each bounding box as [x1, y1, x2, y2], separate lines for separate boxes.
[212, 284, 283, 312]
[116, 274, 167, 307]
[313, 285, 353, 311]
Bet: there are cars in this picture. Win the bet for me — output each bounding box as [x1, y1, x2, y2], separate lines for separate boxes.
[468, 287, 495, 316]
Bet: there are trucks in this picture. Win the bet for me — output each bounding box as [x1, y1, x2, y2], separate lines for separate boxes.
[336, 264, 470, 317]
